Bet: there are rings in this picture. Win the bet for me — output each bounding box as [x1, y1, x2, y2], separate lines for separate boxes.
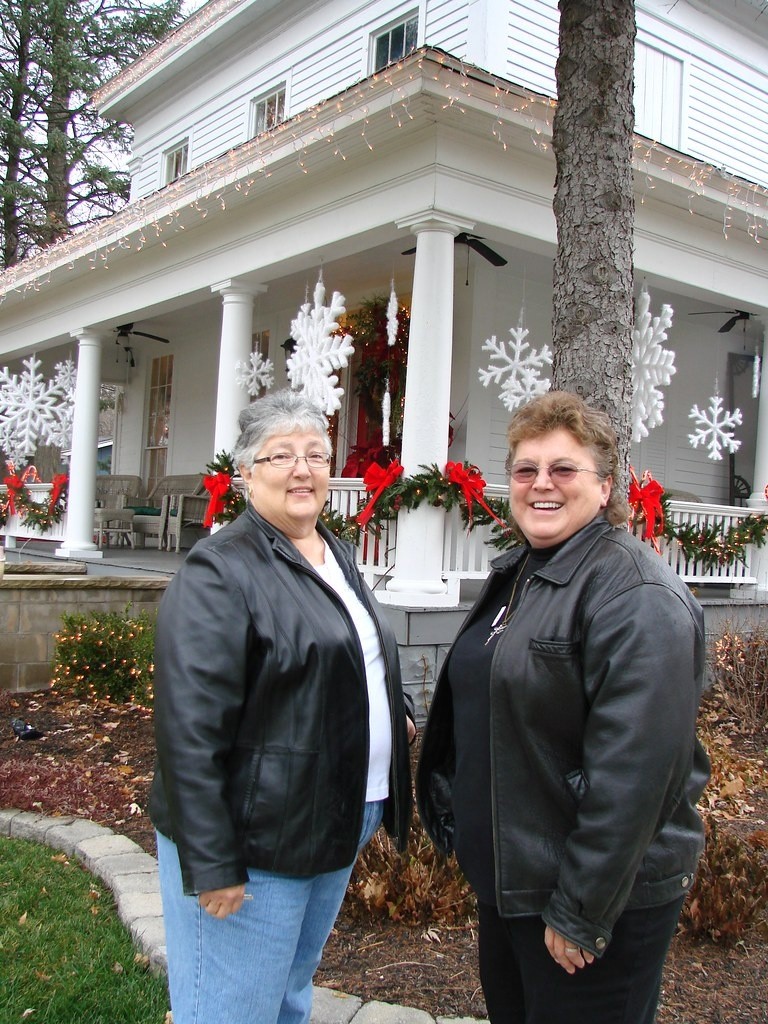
[564, 946, 580, 953]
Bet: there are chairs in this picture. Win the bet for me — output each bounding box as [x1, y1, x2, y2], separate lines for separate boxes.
[94, 475, 212, 554]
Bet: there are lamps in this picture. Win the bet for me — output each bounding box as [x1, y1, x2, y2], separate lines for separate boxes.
[279, 338, 297, 373]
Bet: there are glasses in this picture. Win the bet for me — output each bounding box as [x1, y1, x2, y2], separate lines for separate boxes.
[509, 462, 607, 483]
[253, 452, 334, 468]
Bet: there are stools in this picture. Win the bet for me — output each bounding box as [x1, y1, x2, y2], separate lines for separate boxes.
[94, 508, 135, 550]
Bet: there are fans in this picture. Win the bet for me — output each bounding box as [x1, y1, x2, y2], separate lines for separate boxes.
[401, 232, 508, 286]
[113, 322, 170, 367]
[688, 308, 759, 351]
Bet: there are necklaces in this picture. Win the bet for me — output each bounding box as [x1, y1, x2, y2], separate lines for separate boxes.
[481, 550, 534, 646]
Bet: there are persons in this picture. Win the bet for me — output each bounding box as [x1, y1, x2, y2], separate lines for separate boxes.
[422, 393, 712, 1023]
[148, 386, 416, 1024]
